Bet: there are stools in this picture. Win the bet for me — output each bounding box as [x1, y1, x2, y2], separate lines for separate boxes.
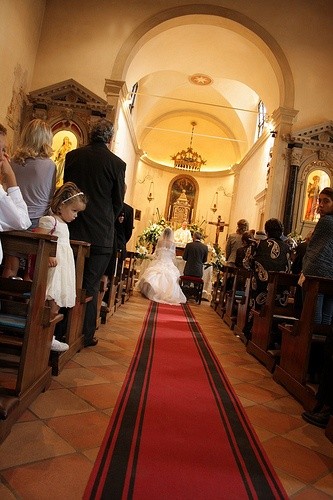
[178, 274, 205, 306]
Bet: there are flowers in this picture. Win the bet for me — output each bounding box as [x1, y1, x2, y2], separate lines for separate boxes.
[135, 220, 166, 247]
[137, 246, 149, 255]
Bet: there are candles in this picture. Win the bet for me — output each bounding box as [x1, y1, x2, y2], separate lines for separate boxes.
[214, 191, 218, 204]
[149, 181, 154, 194]
[191, 208, 194, 220]
[167, 205, 172, 217]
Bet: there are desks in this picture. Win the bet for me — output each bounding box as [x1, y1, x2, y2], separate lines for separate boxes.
[138, 254, 213, 303]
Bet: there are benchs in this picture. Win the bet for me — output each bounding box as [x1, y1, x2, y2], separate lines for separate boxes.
[0, 229, 141, 447]
[208, 264, 333, 443]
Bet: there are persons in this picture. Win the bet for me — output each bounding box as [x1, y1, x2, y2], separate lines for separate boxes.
[305, 175, 321, 221]
[138, 228, 186, 304]
[0, 116, 333, 428]
[182, 232, 208, 298]
[174, 220, 192, 242]
[54, 137, 72, 185]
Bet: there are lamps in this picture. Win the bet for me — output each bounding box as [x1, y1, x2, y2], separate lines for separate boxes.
[168, 121, 208, 178]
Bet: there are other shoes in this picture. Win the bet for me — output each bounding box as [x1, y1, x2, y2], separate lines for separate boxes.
[50, 336, 70, 352]
[85, 337, 98, 347]
[302, 409, 328, 428]
